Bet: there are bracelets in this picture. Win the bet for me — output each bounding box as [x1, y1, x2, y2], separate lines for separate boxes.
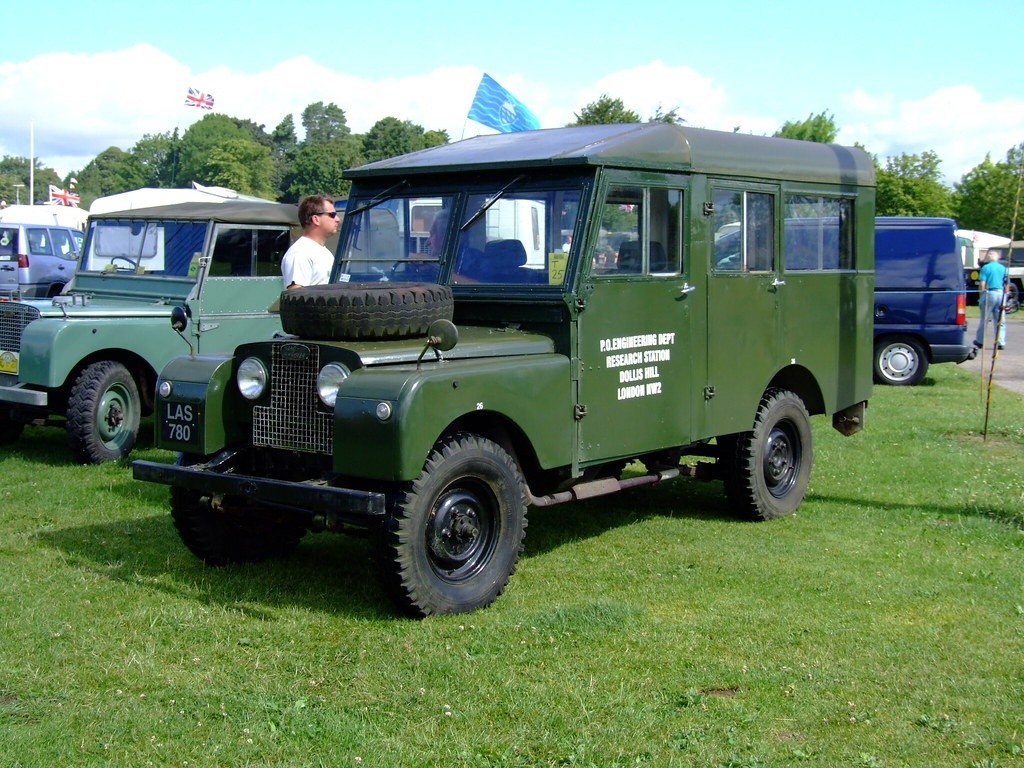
[453, 273, 459, 285]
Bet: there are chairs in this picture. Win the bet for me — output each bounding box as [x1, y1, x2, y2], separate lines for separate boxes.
[616, 239, 667, 272]
[483, 238, 539, 281]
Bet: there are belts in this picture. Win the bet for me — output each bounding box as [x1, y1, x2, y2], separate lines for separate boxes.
[988, 288, 1003, 292]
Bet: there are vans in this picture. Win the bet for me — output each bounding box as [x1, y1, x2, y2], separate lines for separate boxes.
[0, 223, 86, 298]
[713, 217, 979, 386]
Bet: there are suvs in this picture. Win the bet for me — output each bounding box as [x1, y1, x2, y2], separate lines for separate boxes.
[0, 123, 874, 617]
[978, 241, 1024, 312]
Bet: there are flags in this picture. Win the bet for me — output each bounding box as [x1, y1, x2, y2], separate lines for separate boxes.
[49, 185, 79, 208]
[467, 73, 541, 133]
[184, 88, 214, 111]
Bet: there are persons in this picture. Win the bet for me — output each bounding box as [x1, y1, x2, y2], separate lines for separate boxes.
[973, 251, 1011, 350]
[407, 208, 501, 286]
[282, 194, 339, 291]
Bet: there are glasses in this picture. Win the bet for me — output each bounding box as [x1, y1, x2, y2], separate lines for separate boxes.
[312, 212, 337, 219]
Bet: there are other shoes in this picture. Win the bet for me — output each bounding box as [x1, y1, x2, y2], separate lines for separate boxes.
[998, 345, 1005, 350]
[973, 340, 983, 349]
